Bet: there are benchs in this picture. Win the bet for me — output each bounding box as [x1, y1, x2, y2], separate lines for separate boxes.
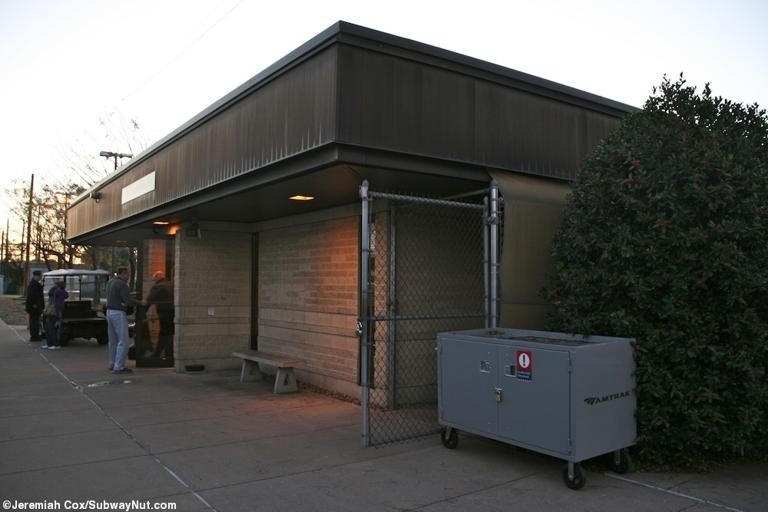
[231, 349, 304, 395]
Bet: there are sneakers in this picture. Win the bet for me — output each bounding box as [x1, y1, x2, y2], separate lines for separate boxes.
[41, 344, 61, 350]
[108, 365, 132, 374]
[31, 335, 41, 341]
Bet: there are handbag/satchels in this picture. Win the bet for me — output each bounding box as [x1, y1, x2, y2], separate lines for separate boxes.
[45, 304, 60, 317]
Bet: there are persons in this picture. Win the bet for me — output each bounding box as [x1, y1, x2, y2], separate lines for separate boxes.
[142, 271, 174, 362]
[40, 279, 69, 350]
[23, 270, 45, 341]
[104, 267, 148, 375]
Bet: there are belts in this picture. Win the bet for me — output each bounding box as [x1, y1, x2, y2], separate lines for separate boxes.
[107, 306, 124, 310]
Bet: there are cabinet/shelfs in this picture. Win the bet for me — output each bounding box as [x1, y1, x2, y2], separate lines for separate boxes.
[432, 322, 638, 461]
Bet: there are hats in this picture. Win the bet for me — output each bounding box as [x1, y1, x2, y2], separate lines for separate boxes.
[33, 271, 41, 276]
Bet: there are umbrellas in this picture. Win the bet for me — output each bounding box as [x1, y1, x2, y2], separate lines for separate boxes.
[41, 267, 110, 301]
